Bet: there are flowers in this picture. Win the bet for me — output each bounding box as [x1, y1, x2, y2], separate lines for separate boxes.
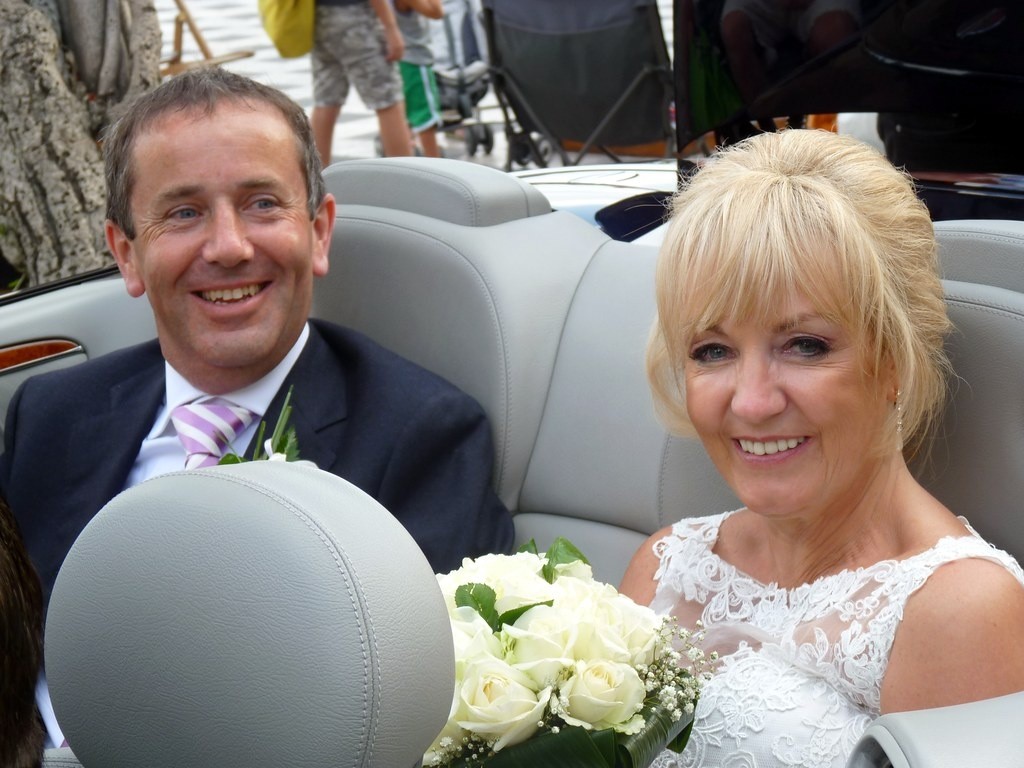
[419, 537, 724, 768]
[208, 385, 318, 467]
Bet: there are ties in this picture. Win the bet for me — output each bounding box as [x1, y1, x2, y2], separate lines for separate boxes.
[61, 403, 260, 749]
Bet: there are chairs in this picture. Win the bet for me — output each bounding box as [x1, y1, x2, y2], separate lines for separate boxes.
[44, 462, 455, 768]
[480, 0, 674, 172]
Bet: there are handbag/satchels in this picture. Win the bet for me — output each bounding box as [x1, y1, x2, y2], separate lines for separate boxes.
[259, 0, 315, 59]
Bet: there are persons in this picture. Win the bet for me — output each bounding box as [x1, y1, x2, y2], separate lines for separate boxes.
[309, 0, 442, 166]
[613, 131, 1024, 768]
[1, 63, 514, 767]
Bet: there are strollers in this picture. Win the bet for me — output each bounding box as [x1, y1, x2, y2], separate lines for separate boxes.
[416, 12, 552, 172]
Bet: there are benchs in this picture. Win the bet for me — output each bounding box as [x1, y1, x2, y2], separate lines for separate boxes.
[309, 156, 1024, 768]
